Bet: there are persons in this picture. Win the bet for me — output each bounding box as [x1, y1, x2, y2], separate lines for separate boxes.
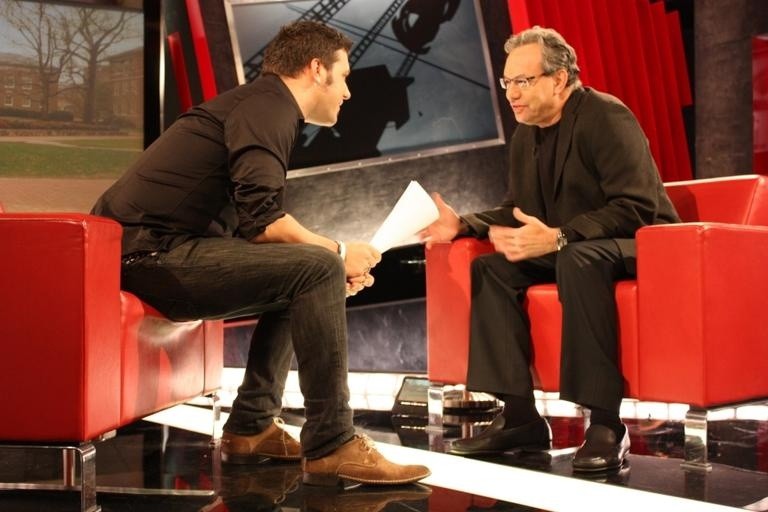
[86, 20, 430, 487]
[414, 23, 687, 475]
[199, 457, 433, 511]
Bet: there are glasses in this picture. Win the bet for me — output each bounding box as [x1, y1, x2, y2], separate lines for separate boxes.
[499, 71, 548, 90]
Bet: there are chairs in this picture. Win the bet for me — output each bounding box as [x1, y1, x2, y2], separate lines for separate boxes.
[0, 204, 225, 511]
[426, 174, 767, 473]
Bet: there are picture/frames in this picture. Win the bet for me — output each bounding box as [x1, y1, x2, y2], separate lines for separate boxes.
[222, 2, 507, 182]
[0, 1, 166, 213]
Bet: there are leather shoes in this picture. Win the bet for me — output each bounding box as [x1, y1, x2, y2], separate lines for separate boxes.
[450, 409, 554, 454]
[222, 418, 304, 467]
[571, 419, 631, 474]
[302, 432, 431, 488]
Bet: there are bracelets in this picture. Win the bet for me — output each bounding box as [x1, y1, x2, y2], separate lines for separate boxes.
[556, 230, 569, 251]
[334, 240, 346, 261]
